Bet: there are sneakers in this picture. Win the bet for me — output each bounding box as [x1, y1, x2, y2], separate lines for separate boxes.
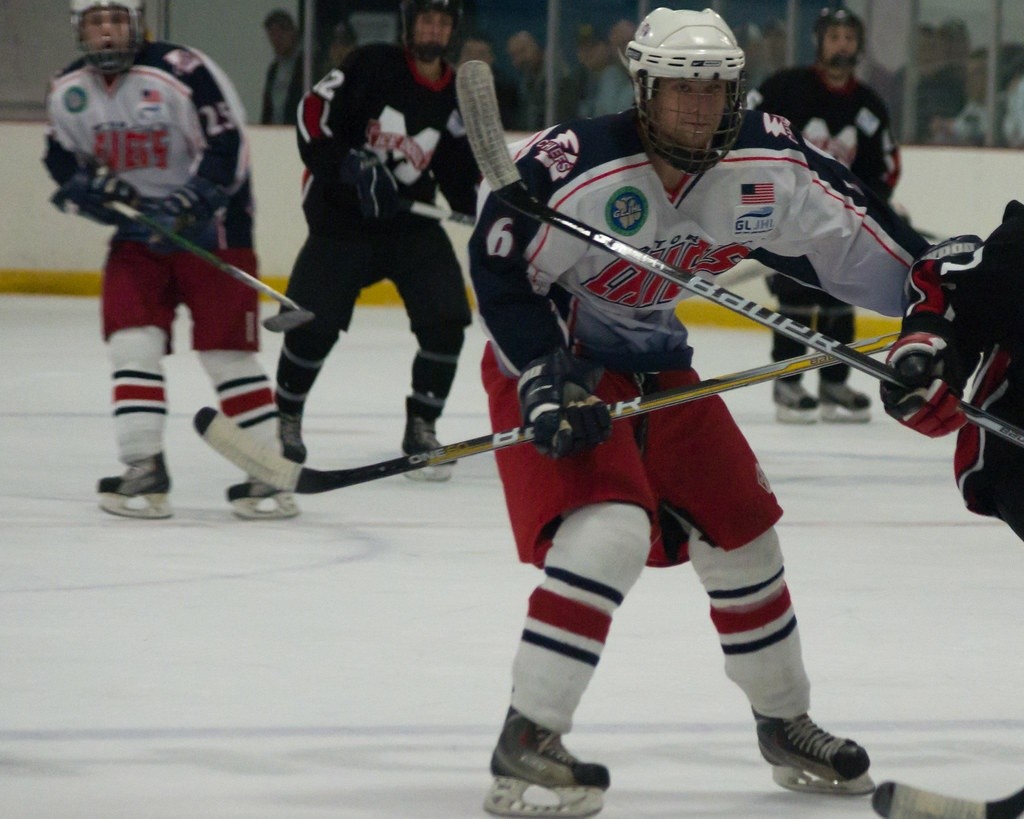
[819, 380, 872, 424]
[771, 377, 819, 425]
[226, 473, 302, 520]
[749, 704, 876, 794]
[277, 405, 305, 462]
[481, 706, 610, 819]
[96, 453, 173, 519]
[404, 398, 455, 481]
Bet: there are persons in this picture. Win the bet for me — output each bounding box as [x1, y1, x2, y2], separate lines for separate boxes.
[274, 0, 484, 475]
[43, 0, 300, 519]
[747, 6, 902, 424]
[258, 8, 1024, 149]
[880, 199, 1024, 541]
[467, 7, 931, 817]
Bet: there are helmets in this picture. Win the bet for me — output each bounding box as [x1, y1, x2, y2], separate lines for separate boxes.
[810, 4, 867, 71]
[626, 5, 750, 175]
[395, 0, 462, 63]
[71, 0, 144, 74]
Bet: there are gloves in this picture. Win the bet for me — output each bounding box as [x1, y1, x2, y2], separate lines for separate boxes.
[515, 348, 613, 460]
[878, 306, 969, 437]
[72, 170, 139, 228]
[350, 147, 402, 223]
[143, 177, 201, 247]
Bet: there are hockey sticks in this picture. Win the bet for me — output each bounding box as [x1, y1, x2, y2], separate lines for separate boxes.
[410, 198, 777, 288]
[452, 56, 1024, 448]
[104, 199, 313, 332]
[189, 332, 901, 497]
